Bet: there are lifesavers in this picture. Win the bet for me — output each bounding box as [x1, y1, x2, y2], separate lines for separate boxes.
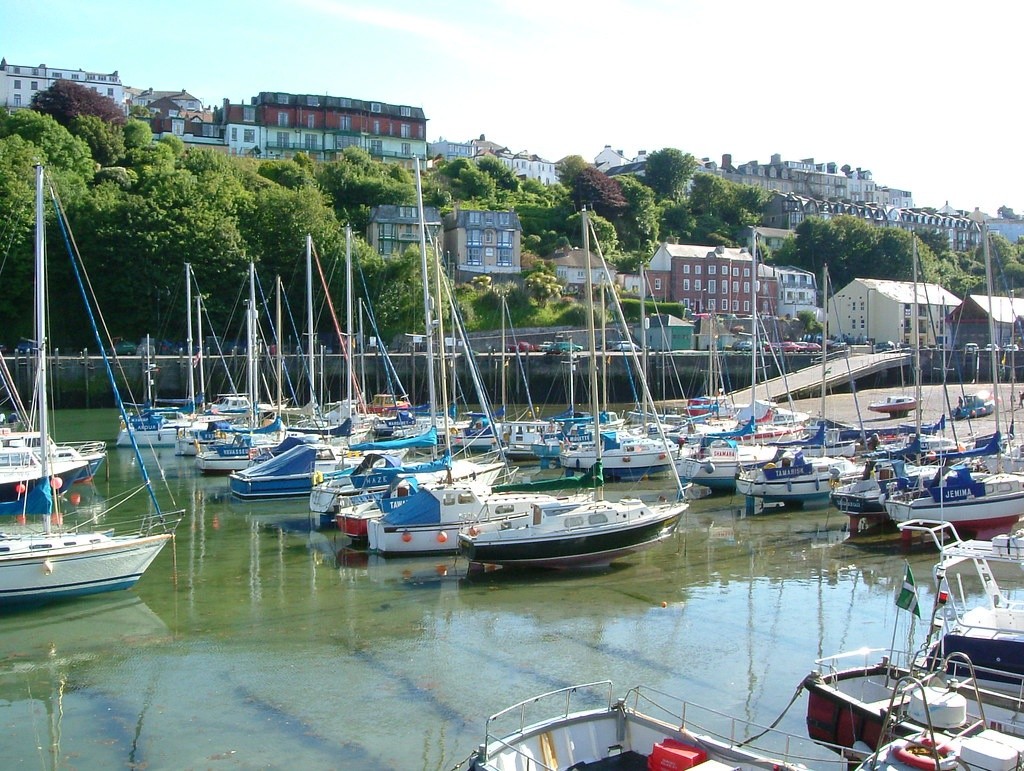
[450, 427, 458, 435]
[312, 470, 324, 485]
[894, 737, 959, 770]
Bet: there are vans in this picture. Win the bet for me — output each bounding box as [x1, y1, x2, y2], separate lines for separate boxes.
[613, 341, 641, 352]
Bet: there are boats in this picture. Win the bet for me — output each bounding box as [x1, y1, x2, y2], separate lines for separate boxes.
[467, 519, 1024, 771]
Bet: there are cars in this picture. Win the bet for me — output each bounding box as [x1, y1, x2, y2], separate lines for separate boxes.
[102, 341, 138, 355]
[734, 341, 821, 353]
[986, 343, 1000, 351]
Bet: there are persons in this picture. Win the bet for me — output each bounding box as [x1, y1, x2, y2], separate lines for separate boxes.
[1018, 391, 1023, 409]
[868, 433, 881, 450]
[958, 396, 963, 407]
[1010, 392, 1016, 406]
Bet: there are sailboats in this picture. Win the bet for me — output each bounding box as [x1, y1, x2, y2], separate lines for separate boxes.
[116, 156, 1024, 566]
[0, 158, 186, 604]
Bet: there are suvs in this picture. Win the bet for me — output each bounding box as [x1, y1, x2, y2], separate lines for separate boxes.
[966, 343, 979, 352]
[548, 342, 581, 352]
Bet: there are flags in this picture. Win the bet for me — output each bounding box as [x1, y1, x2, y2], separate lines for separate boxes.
[896, 564, 921, 621]
[193, 351, 201, 369]
